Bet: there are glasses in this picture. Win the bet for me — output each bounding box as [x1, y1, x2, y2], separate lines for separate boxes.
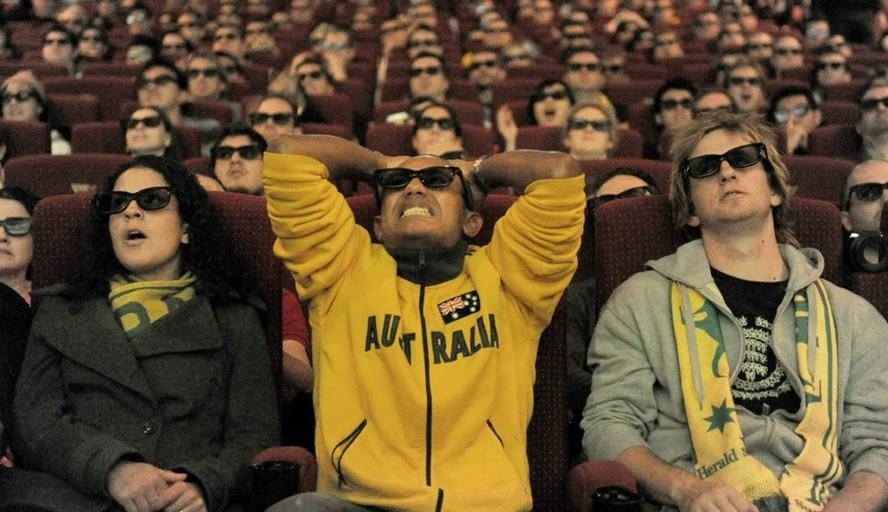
[845, 182, 888, 211]
[470, 18, 529, 71]
[571, 64, 622, 76]
[1, 217, 36, 236]
[209, 144, 258, 160]
[860, 96, 888, 113]
[371, 167, 473, 214]
[571, 117, 610, 130]
[119, 114, 169, 129]
[133, 63, 235, 91]
[586, 183, 658, 215]
[679, 142, 773, 194]
[749, 39, 848, 71]
[46, 37, 101, 44]
[1, 91, 36, 102]
[249, 111, 292, 128]
[88, 186, 177, 216]
[661, 75, 815, 122]
[407, 37, 454, 134]
[299, 71, 323, 81]
[535, 91, 565, 102]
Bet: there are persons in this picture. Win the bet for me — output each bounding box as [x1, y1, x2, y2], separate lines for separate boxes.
[580, 112, 886, 512]
[262, 138, 586, 511]
[1, 0, 887, 373]
[0, 156, 291, 512]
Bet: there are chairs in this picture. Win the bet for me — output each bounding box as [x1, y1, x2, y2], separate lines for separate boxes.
[0, 0, 888, 511]
